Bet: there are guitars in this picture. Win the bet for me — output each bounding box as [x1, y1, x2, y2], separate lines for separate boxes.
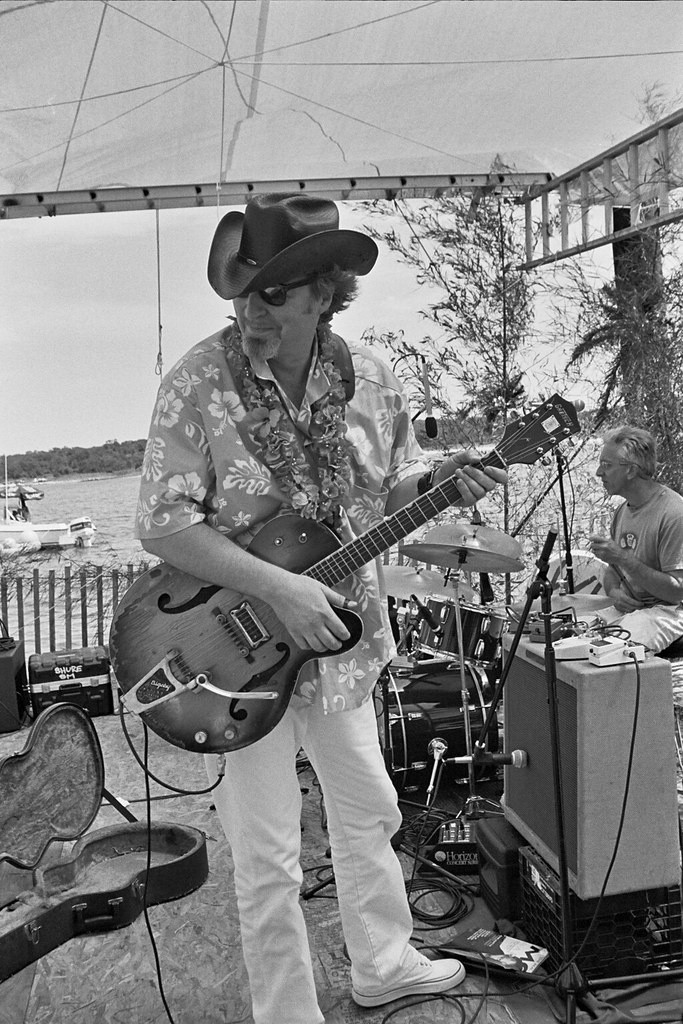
[108, 390, 585, 756]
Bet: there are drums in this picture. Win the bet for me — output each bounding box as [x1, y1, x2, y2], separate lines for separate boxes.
[372, 659, 499, 791]
[413, 593, 508, 668]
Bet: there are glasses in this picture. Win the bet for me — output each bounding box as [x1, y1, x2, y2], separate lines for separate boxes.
[598, 460, 627, 469]
[233, 283, 293, 306]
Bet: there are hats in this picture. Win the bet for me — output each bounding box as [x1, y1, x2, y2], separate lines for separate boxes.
[207, 190, 379, 301]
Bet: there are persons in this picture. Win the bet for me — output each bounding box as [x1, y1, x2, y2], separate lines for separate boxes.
[564, 426, 683, 660]
[132, 191, 510, 1024]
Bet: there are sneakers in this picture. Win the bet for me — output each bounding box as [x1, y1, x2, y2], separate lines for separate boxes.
[349, 958, 479, 1010]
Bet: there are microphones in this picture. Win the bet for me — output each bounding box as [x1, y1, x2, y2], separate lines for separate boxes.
[410, 594, 445, 638]
[473, 510, 495, 603]
[569, 399, 585, 412]
[420, 355, 438, 439]
[445, 749, 528, 769]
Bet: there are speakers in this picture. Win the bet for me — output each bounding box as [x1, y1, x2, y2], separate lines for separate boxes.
[503, 635, 681, 896]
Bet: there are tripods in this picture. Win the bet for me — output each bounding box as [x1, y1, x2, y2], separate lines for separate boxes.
[465, 530, 683, 1024]
[302, 549, 504, 901]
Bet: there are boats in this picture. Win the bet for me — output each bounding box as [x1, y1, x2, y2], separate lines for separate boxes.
[0, 476, 97, 552]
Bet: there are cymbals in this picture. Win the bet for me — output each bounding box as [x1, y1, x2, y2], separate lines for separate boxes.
[424, 524, 523, 562]
[509, 593, 616, 615]
[397, 544, 526, 574]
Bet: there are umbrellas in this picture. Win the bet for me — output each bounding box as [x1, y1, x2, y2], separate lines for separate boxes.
[0, 484, 45, 515]
